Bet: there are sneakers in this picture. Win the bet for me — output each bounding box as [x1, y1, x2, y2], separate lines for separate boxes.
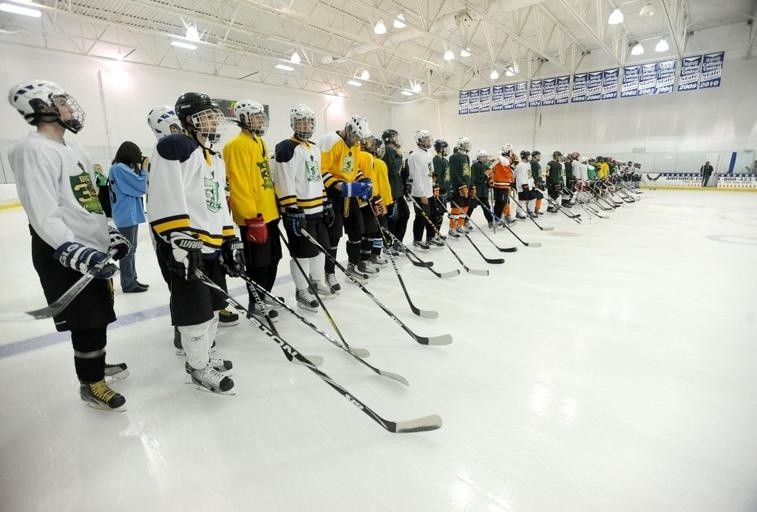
[413, 201, 575, 251]
[80, 379, 125, 409]
[175, 243, 409, 392]
[105, 363, 126, 375]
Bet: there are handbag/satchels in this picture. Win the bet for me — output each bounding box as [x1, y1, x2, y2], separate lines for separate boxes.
[98, 176, 111, 217]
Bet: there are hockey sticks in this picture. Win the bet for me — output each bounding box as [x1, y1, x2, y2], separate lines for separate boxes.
[232, 240, 325, 368]
[194, 225, 452, 432]
[25, 246, 117, 320]
[542, 179, 643, 222]
[196, 269, 442, 433]
[299, 225, 452, 346]
[379, 191, 541, 278]
[275, 225, 372, 359]
[363, 183, 439, 320]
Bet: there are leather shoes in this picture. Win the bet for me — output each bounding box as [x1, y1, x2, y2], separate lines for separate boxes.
[136, 282, 149, 287]
[133, 285, 147, 292]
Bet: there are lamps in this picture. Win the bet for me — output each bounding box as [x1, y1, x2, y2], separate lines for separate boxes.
[374, 18, 388, 35]
[656, 15, 670, 53]
[361, 69, 370, 80]
[506, 66, 516, 77]
[346, 78, 362, 88]
[185, 24, 202, 41]
[629, 43, 644, 55]
[1, 3, 43, 17]
[609, 1, 623, 26]
[443, 47, 455, 60]
[394, 11, 407, 27]
[168, 39, 197, 50]
[290, 49, 301, 63]
[460, 46, 471, 56]
[402, 90, 413, 96]
[414, 83, 422, 92]
[490, 70, 498, 79]
[640, 3, 656, 19]
[275, 61, 293, 72]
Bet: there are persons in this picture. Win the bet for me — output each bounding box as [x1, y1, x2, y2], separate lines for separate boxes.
[108, 141, 148, 294]
[222, 100, 284, 323]
[317, 115, 373, 297]
[147, 91, 245, 396]
[359, 130, 476, 275]
[545, 151, 639, 213]
[274, 104, 334, 312]
[148, 107, 183, 137]
[91, 164, 111, 225]
[8, 80, 131, 413]
[464, 145, 545, 232]
[700, 161, 713, 186]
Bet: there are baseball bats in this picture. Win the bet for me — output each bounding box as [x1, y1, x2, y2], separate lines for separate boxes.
[214, 251, 410, 388]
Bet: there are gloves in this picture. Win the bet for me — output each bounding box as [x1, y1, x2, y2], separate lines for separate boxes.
[108, 225, 133, 261]
[341, 178, 372, 200]
[54, 242, 117, 278]
[405, 184, 529, 199]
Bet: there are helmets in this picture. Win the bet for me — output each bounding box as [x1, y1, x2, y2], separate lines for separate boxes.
[382, 129, 399, 142]
[147, 92, 386, 159]
[9, 80, 85, 133]
[415, 129, 579, 159]
